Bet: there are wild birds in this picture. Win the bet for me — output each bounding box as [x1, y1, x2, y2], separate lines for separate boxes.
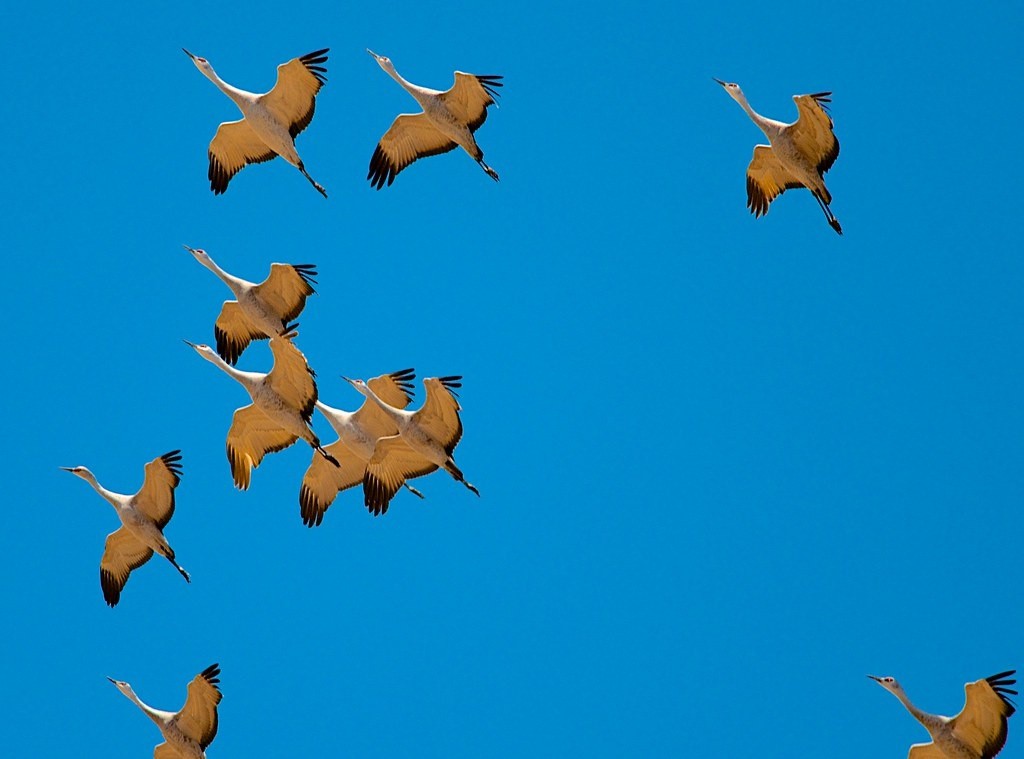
[711, 76, 844, 238]
[340, 375, 481, 518]
[366, 48, 504, 192]
[181, 323, 342, 491]
[299, 367, 417, 528]
[866, 670, 1019, 759]
[59, 447, 192, 610]
[184, 245, 319, 367]
[104, 662, 225, 759]
[182, 48, 330, 199]
